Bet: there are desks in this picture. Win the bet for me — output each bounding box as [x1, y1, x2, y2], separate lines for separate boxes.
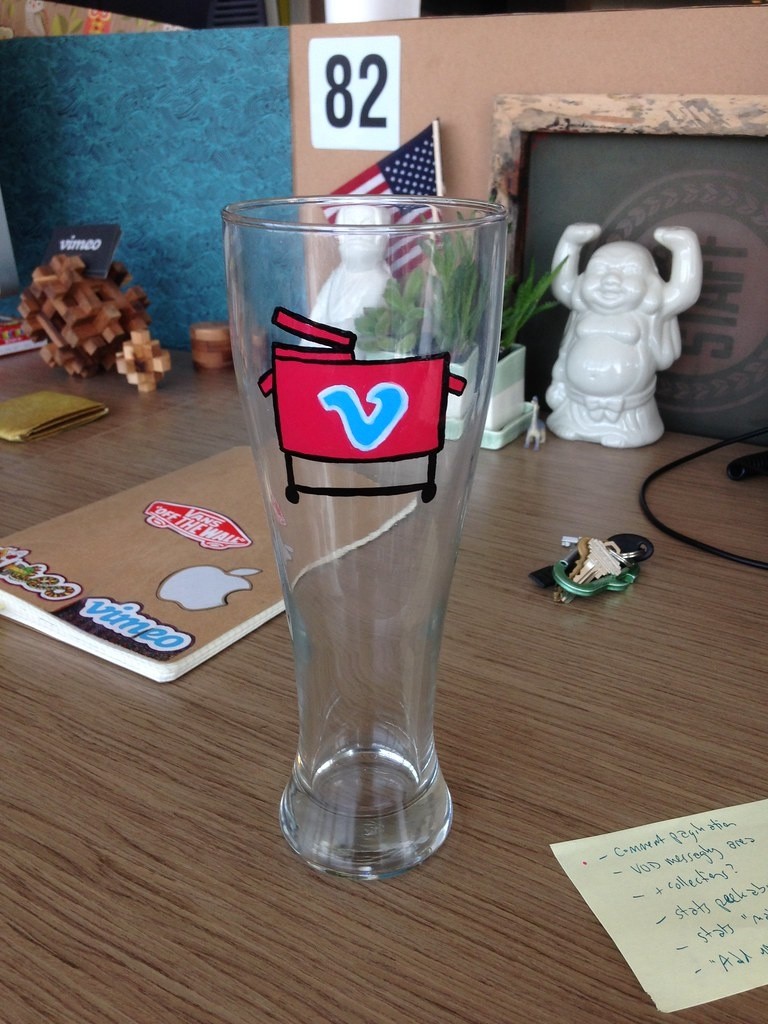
[0, 344, 767, 1024]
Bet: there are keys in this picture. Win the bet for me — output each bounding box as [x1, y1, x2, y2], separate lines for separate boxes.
[528, 534, 656, 606]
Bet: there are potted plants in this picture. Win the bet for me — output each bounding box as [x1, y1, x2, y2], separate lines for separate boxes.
[355, 188, 570, 452]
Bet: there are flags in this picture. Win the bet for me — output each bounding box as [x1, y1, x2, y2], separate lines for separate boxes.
[321, 118, 447, 277]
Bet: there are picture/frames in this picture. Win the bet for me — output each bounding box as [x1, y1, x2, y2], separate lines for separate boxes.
[486, 95, 767, 444]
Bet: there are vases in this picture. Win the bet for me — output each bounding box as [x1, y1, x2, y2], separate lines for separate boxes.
[216, 199, 511, 879]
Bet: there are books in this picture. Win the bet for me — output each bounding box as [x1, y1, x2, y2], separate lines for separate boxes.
[0, 297, 48, 357]
[0, 444, 419, 684]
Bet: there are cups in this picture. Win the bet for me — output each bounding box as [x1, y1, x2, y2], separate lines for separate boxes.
[221, 196, 508, 875]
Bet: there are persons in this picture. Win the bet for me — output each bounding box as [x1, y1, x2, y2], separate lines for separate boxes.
[545, 223, 705, 450]
